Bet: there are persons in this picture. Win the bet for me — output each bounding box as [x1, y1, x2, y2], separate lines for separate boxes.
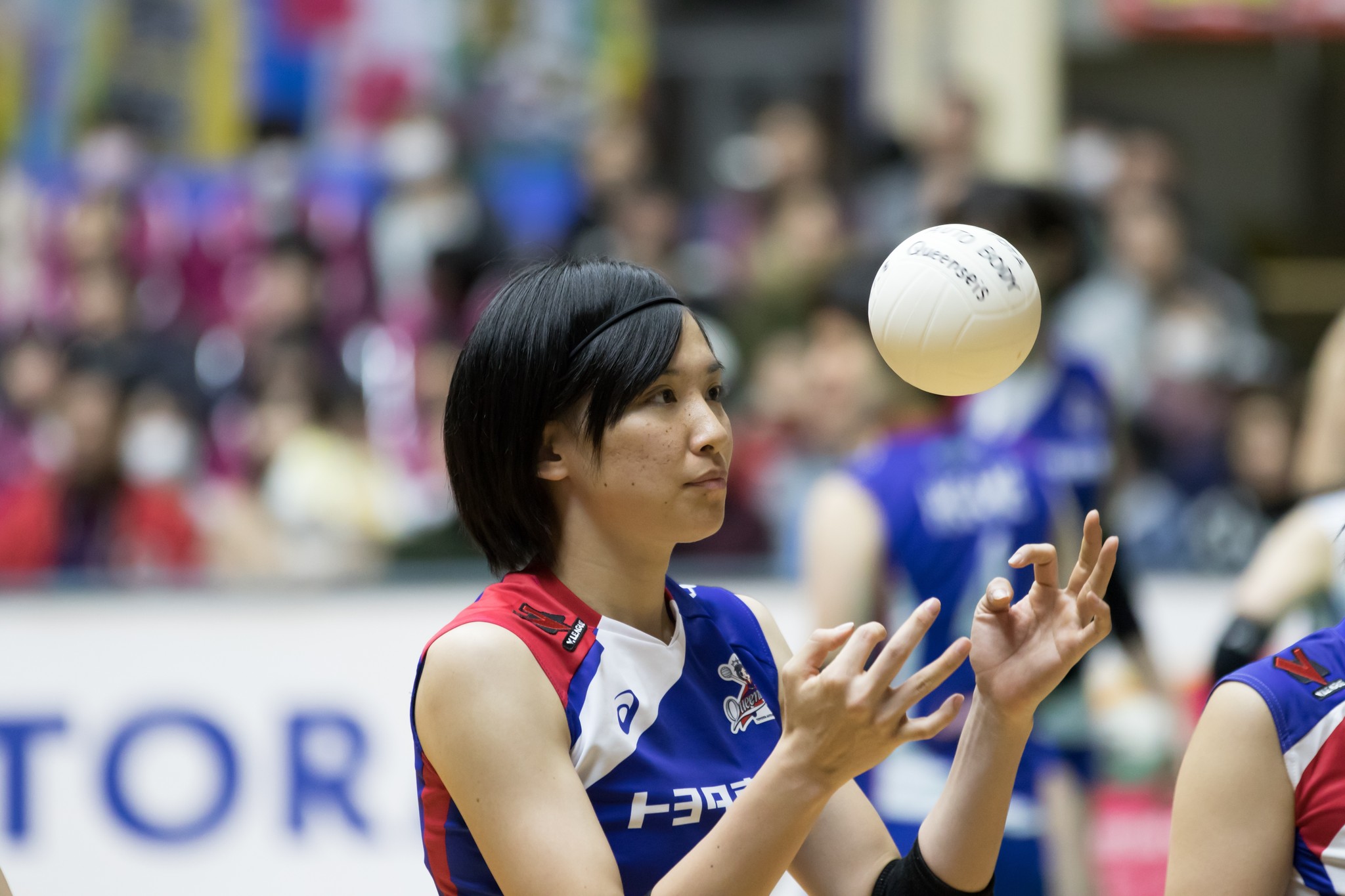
[1164, 619, 1345, 896]
[0, 0, 1345, 896]
[408, 255, 1120, 896]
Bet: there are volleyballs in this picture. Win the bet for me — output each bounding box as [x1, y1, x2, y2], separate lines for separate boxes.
[866, 221, 1043, 397]
